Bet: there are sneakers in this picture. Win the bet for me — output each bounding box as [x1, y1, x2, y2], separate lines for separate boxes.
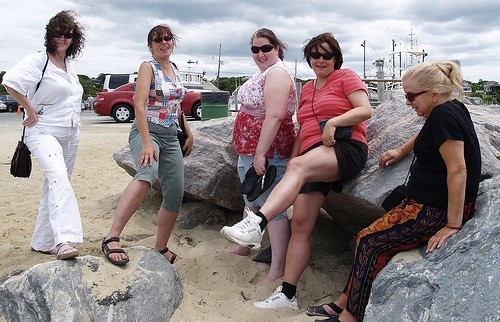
[253, 285, 299, 310]
[220, 206, 268, 251]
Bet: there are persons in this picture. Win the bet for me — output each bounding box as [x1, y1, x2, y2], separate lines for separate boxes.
[87, 94, 94, 110]
[103, 25, 193, 264]
[221, 32, 372, 310]
[2, 10, 83, 259]
[225, 28, 297, 284]
[306, 61, 481, 322]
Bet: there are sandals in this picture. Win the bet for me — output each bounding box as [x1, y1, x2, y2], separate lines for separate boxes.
[306, 302, 344, 317]
[101, 236, 130, 265]
[54, 242, 79, 260]
[154, 246, 182, 265]
[315, 316, 341, 322]
[31, 243, 57, 255]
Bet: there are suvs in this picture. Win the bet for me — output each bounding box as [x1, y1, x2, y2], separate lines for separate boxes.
[0, 94, 21, 113]
[103, 73, 212, 94]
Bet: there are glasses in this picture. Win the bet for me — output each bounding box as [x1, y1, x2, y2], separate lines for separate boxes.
[251, 44, 275, 54]
[152, 35, 172, 43]
[404, 90, 428, 102]
[309, 51, 334, 60]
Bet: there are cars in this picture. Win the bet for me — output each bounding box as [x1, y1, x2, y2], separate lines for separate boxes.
[92, 82, 204, 123]
[0, 101, 7, 112]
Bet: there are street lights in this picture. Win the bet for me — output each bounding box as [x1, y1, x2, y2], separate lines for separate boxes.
[360, 40, 366, 79]
[392, 39, 397, 79]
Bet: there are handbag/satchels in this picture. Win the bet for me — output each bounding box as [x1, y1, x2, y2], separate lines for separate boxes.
[380, 185, 407, 213]
[8, 140, 32, 178]
[176, 129, 190, 158]
[319, 118, 353, 141]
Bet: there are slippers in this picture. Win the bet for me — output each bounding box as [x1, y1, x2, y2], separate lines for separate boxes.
[246, 164, 278, 202]
[239, 157, 270, 195]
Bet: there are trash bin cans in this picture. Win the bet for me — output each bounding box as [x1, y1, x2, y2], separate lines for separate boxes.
[199, 90, 232, 123]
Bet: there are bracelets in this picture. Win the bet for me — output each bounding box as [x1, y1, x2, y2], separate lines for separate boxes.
[446, 223, 462, 229]
